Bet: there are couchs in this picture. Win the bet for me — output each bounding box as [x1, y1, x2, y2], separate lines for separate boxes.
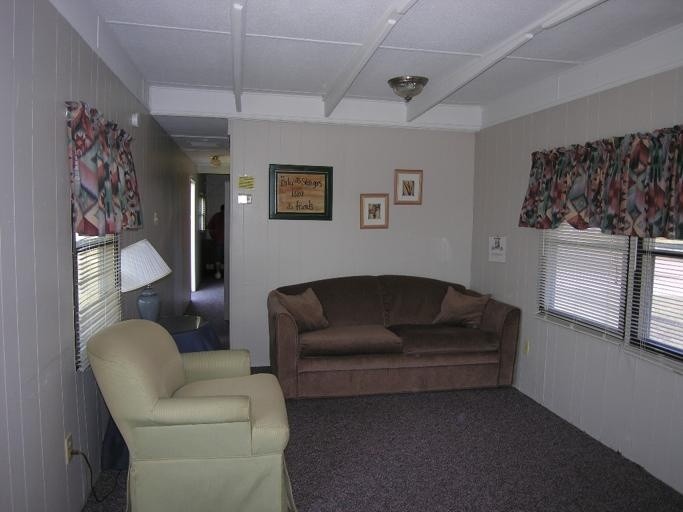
[266, 274, 520, 399]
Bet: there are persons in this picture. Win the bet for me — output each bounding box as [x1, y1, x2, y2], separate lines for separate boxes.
[208, 204, 224, 280]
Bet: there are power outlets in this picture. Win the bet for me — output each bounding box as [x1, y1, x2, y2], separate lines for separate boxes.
[65, 433, 74, 464]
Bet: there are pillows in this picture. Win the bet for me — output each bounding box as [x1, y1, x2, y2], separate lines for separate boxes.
[431, 285, 492, 329]
[271, 287, 328, 333]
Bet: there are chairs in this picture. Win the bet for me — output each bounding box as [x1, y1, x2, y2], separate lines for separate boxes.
[84, 320, 290, 512]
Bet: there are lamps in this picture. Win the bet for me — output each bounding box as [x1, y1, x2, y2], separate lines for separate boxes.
[387, 76, 429, 103]
[120, 238, 172, 322]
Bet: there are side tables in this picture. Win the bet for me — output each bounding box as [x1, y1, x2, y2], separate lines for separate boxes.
[156, 314, 220, 353]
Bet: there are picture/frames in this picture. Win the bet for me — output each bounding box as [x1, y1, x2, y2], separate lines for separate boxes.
[393, 169, 423, 205]
[268, 164, 333, 220]
[359, 192, 390, 229]
